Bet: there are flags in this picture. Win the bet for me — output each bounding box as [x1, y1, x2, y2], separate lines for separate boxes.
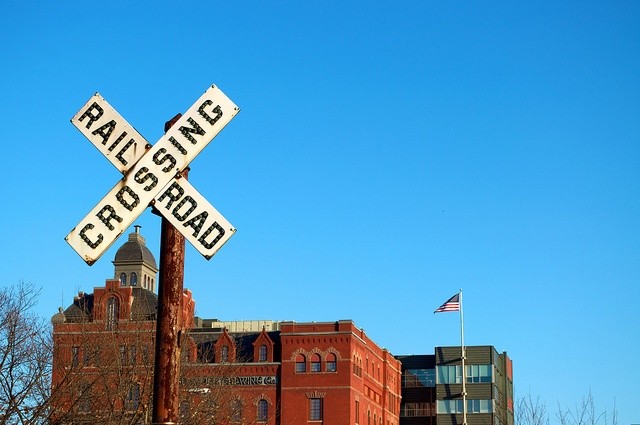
[432, 293, 461, 316]
